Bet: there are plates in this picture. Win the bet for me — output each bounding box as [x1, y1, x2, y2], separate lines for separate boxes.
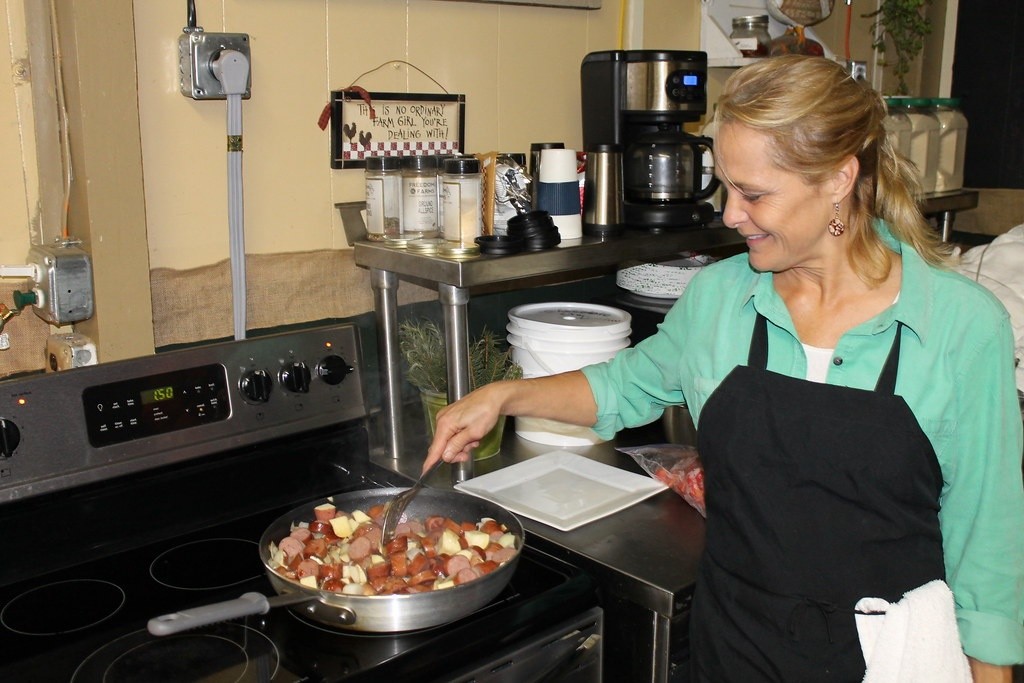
[474, 211, 561, 256]
[453, 450, 669, 531]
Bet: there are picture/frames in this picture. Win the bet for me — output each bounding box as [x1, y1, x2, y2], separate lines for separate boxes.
[330, 89, 466, 170]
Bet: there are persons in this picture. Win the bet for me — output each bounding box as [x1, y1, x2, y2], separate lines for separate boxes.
[420, 54, 1024, 683]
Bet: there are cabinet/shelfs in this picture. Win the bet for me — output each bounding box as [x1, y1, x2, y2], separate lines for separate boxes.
[353, 184, 979, 683]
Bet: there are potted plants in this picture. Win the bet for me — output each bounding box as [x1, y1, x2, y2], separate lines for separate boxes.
[401, 314, 524, 461]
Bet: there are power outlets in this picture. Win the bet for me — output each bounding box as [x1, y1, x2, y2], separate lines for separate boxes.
[180, 29, 254, 103]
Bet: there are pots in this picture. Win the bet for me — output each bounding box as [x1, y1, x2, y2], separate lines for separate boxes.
[146, 485, 526, 637]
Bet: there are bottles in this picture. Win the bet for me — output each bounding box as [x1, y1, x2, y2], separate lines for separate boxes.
[362, 153, 533, 254]
[880, 96, 969, 195]
[729, 15, 772, 58]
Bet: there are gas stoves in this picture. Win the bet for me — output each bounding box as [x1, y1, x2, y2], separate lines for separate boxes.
[0, 321, 601, 683]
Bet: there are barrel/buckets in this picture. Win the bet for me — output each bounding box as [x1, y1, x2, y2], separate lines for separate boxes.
[506, 302, 632, 446]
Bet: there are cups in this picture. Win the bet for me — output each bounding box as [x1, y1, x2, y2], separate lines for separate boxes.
[538, 149, 583, 240]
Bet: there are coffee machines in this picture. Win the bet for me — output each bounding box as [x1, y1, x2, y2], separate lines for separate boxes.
[580, 51, 722, 234]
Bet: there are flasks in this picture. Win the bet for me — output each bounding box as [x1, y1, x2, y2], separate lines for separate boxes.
[584, 144, 624, 237]
